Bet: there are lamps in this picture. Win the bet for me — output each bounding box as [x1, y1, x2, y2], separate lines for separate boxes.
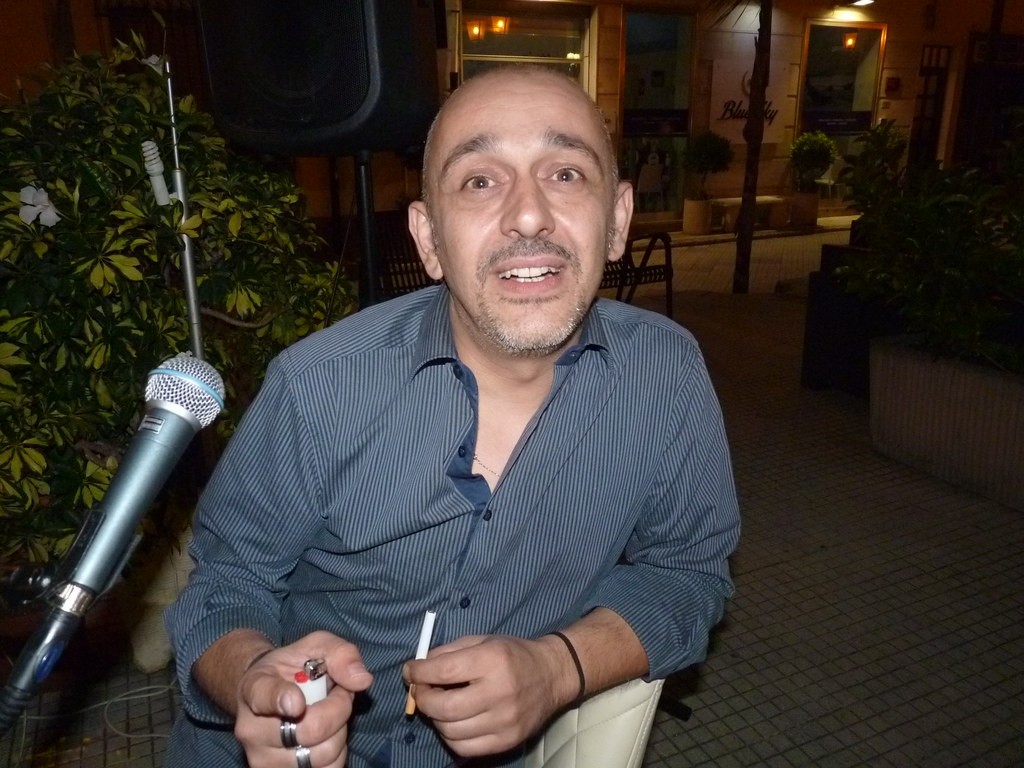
[848, 0, 874, 6]
[842, 29, 858, 49]
[467, 19, 487, 43]
[489, 14, 513, 37]
[139, 53, 203, 360]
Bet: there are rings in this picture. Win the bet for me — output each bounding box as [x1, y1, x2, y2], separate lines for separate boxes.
[279, 718, 299, 747]
[296, 747, 311, 768]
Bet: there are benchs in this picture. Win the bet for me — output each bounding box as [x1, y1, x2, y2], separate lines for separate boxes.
[346, 209, 676, 320]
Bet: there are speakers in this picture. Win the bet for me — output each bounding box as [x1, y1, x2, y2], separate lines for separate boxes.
[191, 0, 421, 164]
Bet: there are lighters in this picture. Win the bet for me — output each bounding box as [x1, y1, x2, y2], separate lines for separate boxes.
[294, 658, 327, 705]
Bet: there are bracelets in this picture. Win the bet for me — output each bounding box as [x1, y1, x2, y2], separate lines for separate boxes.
[545, 630, 586, 709]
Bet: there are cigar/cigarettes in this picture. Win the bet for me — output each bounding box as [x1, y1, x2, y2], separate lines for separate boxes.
[405, 609, 437, 715]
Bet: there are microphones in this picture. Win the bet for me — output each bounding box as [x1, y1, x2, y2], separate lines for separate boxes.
[0, 356, 226, 738]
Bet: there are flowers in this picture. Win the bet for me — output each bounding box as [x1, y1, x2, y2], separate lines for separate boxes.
[18, 185, 62, 227]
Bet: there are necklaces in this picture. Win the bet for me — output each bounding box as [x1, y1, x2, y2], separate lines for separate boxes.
[474, 455, 500, 479]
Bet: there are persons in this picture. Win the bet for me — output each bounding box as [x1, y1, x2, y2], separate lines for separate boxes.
[164, 65, 742, 768]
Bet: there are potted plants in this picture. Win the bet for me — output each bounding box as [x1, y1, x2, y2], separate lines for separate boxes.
[830, 113, 1024, 511]
[680, 129, 736, 237]
[787, 131, 839, 229]
